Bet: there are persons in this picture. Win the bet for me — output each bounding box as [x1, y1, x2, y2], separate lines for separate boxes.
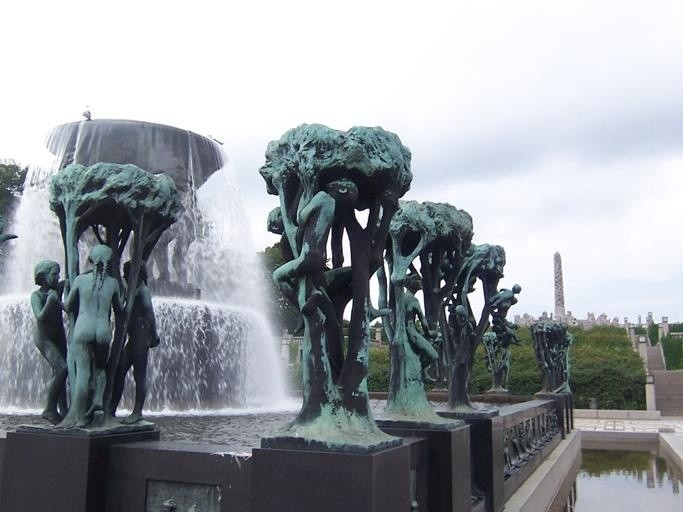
[30, 260, 68, 424]
[120, 259, 160, 424]
[61, 244, 125, 428]
[266, 178, 572, 390]
